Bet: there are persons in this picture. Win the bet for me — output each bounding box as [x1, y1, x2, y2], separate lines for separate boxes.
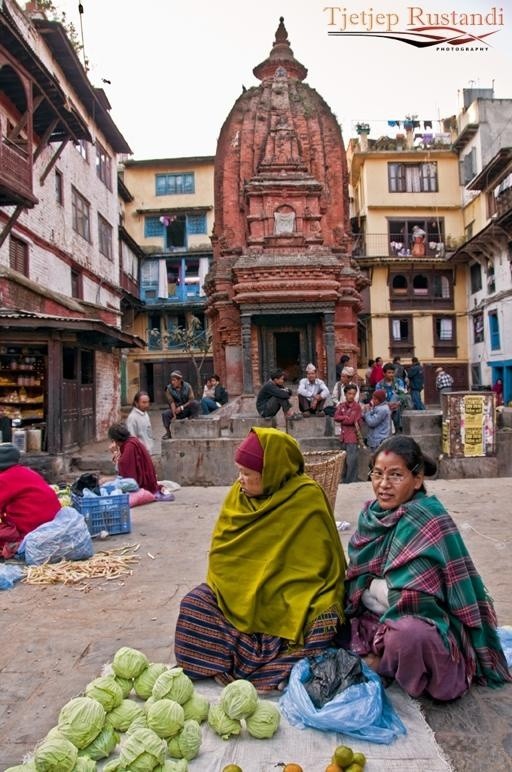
[201, 375, 228, 415]
[492, 379, 503, 406]
[203, 378, 215, 398]
[345, 436, 512, 702]
[331, 356, 424, 483]
[162, 370, 200, 439]
[0, 444, 61, 558]
[256, 368, 303, 420]
[126, 391, 154, 456]
[435, 367, 454, 410]
[108, 424, 158, 494]
[298, 363, 330, 418]
[175, 426, 348, 700]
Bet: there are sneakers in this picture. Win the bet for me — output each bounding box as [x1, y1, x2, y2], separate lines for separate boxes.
[162, 434, 170, 439]
[315, 410, 326, 416]
[303, 411, 310, 417]
[288, 413, 303, 420]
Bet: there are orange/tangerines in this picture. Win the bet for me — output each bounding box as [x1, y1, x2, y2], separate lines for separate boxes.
[221, 738, 366, 772]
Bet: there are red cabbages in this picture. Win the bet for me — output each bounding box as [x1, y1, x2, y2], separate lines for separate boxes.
[31, 646, 280, 772]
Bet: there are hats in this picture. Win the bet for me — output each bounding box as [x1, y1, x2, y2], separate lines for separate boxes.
[306, 363, 316, 372]
[1, 444, 20, 470]
[373, 390, 386, 403]
[171, 370, 182, 379]
[234, 430, 264, 473]
[343, 366, 354, 376]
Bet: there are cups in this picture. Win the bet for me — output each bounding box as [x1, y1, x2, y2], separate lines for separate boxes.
[10, 361, 33, 371]
[18, 377, 36, 384]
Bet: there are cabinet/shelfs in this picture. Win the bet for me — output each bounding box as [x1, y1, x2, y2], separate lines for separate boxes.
[1, 341, 48, 424]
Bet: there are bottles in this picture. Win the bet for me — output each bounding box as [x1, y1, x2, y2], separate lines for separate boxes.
[19, 386, 26, 403]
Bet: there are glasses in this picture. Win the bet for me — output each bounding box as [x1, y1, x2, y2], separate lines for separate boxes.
[369, 465, 418, 484]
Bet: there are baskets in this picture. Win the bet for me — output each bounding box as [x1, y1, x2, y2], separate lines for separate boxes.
[302, 450, 347, 513]
[71, 487, 131, 536]
